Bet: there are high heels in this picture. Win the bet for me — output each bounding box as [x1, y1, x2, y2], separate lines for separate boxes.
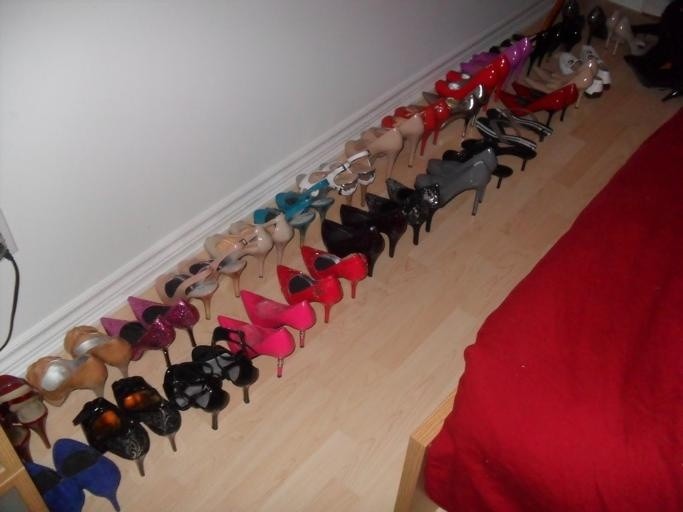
[622, 54, 683, 101]
[612, 18, 658, 56]
[344, 8, 614, 218]
[0, 289, 318, 512]
[605, 9, 621, 48]
[153, 151, 441, 325]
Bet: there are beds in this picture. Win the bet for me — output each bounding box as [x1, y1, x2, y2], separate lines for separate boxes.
[390, 106, 682, 511]
